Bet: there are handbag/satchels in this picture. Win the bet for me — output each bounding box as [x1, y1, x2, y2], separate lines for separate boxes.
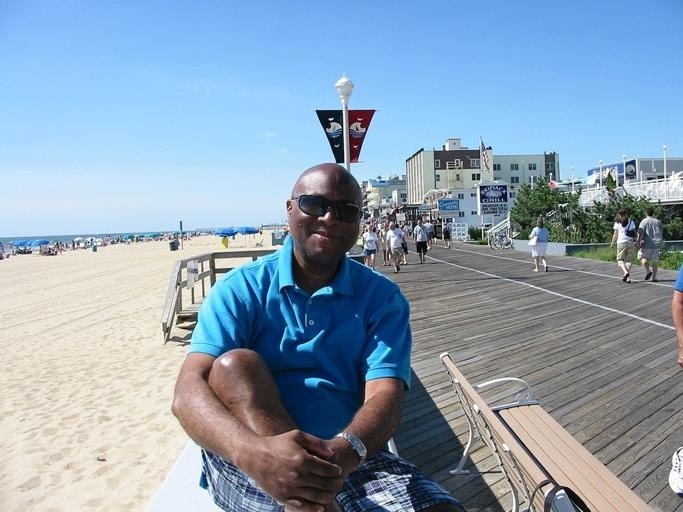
[528, 236, 538, 246]
[625, 229, 635, 237]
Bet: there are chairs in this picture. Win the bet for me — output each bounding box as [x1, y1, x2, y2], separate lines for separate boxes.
[254, 239, 263, 248]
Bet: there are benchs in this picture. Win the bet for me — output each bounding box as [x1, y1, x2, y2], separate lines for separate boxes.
[432, 347, 661, 512]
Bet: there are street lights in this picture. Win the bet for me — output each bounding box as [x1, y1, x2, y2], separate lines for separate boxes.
[529, 174, 533, 190]
[570, 166, 575, 190]
[622, 155, 626, 186]
[548, 171, 552, 181]
[598, 159, 603, 187]
[661, 144, 669, 183]
[334, 70, 354, 172]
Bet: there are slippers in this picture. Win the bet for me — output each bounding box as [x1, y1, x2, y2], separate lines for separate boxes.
[380, 263, 425, 274]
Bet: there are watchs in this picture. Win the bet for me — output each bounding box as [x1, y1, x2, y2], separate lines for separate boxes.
[336, 432, 367, 470]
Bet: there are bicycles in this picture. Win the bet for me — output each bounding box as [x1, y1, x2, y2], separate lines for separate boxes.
[489, 232, 513, 251]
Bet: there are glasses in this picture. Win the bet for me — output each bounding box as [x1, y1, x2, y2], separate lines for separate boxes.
[292, 195, 361, 223]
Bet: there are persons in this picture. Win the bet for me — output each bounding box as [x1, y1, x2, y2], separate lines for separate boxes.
[668, 261, 683, 496]
[171, 163, 467, 512]
[611, 209, 638, 284]
[362, 214, 452, 275]
[529, 218, 549, 273]
[0, 228, 288, 262]
[635, 207, 664, 282]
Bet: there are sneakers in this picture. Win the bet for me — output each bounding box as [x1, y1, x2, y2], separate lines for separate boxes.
[532, 265, 548, 273]
[623, 272, 658, 283]
[669, 446, 683, 494]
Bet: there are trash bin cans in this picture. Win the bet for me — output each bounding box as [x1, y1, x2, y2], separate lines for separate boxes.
[169, 241, 179, 251]
[92, 246, 97, 251]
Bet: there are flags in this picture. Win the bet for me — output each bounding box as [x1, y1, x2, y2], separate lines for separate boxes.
[481, 141, 490, 172]
